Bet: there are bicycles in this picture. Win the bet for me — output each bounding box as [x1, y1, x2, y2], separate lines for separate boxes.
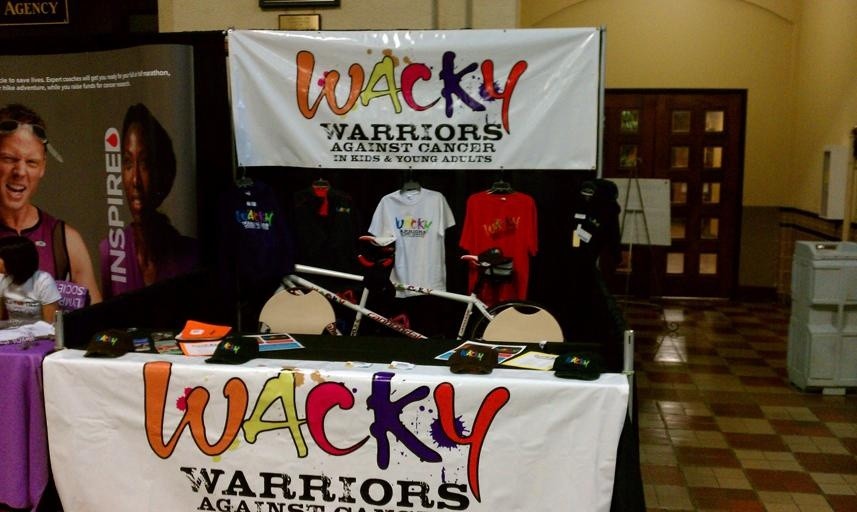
[261, 239, 567, 352]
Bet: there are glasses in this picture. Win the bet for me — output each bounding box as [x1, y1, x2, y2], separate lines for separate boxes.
[0, 120, 48, 144]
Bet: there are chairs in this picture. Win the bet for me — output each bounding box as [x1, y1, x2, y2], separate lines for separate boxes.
[47, 308, 66, 352]
[481, 304, 568, 345]
[256, 289, 338, 337]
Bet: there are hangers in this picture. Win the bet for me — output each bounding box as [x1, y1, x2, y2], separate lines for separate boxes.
[234, 164, 254, 188]
[486, 165, 514, 195]
[577, 167, 598, 199]
[400, 166, 424, 194]
[311, 165, 330, 190]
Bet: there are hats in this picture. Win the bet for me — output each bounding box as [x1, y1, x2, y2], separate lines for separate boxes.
[448, 347, 496, 376]
[478, 247, 512, 265]
[552, 343, 602, 381]
[204, 337, 259, 365]
[83, 329, 136, 359]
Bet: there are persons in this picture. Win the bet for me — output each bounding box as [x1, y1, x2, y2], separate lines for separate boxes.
[0, 103, 105, 308]
[99, 102, 201, 300]
[0, 234, 64, 330]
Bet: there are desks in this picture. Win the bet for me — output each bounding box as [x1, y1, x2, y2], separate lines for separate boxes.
[1, 339, 52, 511]
[41, 336, 644, 511]
